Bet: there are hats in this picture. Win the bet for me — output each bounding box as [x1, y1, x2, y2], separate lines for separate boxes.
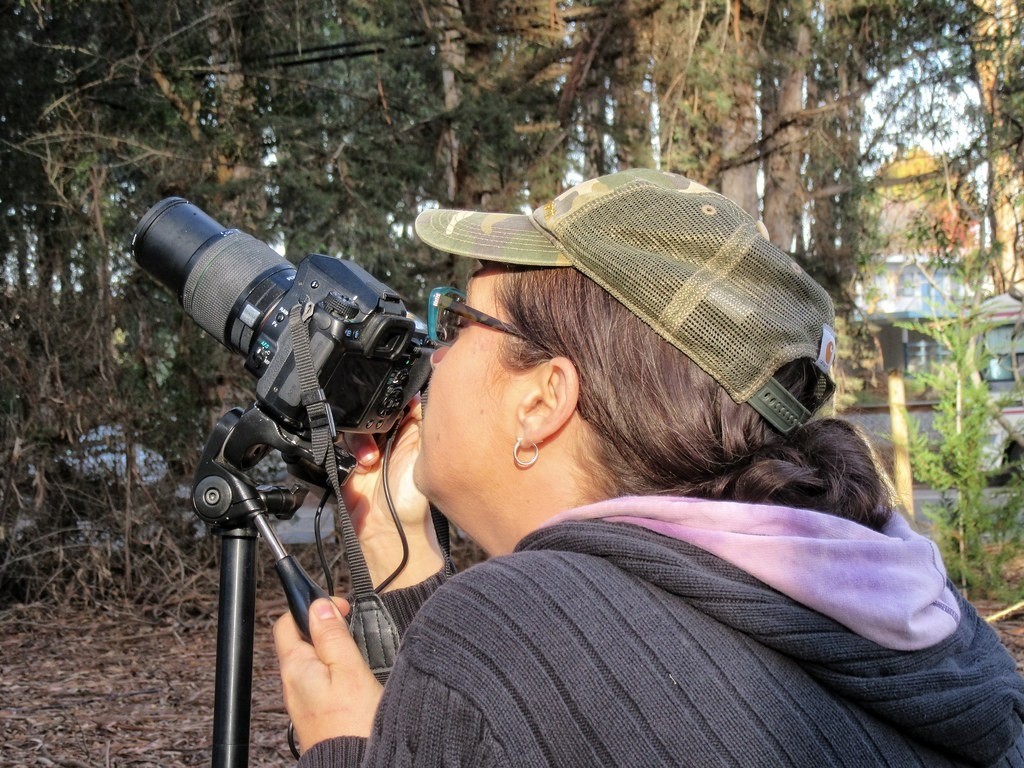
[416, 169, 838, 433]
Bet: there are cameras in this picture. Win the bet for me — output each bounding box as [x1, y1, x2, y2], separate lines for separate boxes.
[130, 195, 437, 436]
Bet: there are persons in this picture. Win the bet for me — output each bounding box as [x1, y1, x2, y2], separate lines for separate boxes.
[273, 167, 1024, 768]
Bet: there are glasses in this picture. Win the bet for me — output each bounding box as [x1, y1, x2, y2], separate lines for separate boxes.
[427, 287, 586, 420]
